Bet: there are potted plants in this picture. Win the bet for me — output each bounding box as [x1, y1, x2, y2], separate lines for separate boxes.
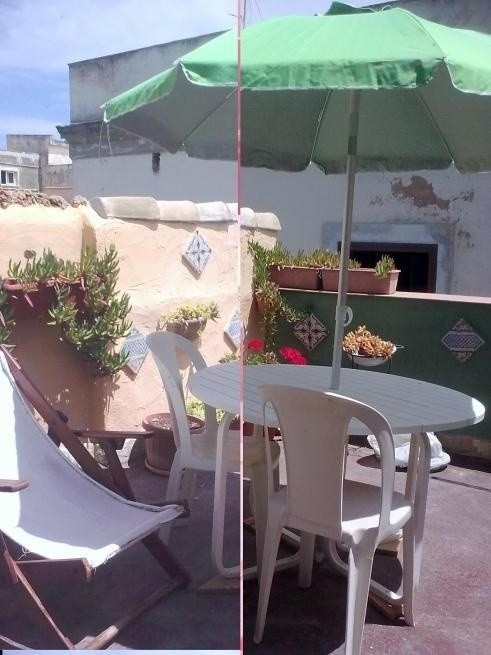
[0, 243, 137, 379]
[160, 302, 220, 337]
[244, 234, 402, 316]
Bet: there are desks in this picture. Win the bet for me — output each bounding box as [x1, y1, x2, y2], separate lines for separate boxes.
[184, 357, 488, 630]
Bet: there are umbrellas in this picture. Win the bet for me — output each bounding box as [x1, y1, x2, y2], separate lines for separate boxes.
[96, 0, 491, 393]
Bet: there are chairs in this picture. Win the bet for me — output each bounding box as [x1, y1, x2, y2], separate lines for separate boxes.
[143, 324, 421, 654]
[0, 330, 201, 653]
